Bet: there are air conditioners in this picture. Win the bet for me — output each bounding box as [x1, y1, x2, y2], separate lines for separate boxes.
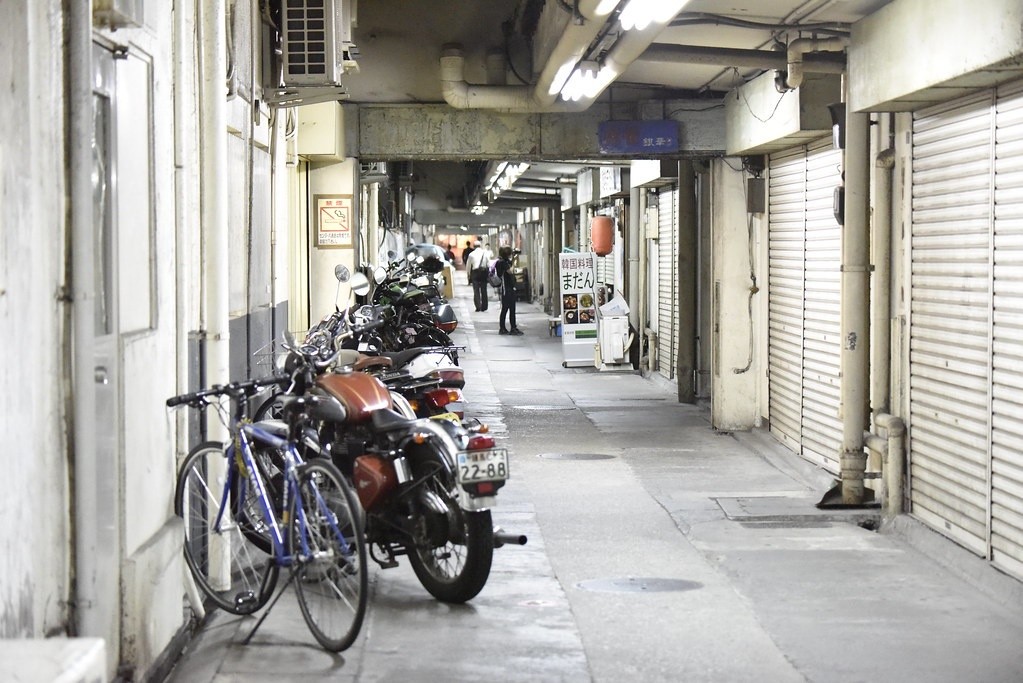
[272, 0, 345, 86]
[360, 161, 388, 174]
[396, 160, 416, 186]
[598, 315, 630, 363]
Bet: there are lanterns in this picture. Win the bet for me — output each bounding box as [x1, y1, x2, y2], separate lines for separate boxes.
[592, 214, 612, 257]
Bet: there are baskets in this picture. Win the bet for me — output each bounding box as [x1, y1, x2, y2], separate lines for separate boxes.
[253, 330, 309, 377]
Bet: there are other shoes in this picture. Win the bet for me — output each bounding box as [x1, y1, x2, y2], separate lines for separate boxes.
[499, 328, 510, 334]
[510, 328, 524, 335]
[482, 306, 487, 310]
[475, 308, 484, 312]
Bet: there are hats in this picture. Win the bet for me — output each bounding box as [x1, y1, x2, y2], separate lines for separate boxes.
[499, 246, 512, 257]
[473, 241, 481, 245]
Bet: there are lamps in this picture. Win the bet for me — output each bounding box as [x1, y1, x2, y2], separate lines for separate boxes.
[560, 61, 600, 101]
[618, 0, 670, 30]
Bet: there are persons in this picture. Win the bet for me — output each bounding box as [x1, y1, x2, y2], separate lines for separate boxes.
[462, 241, 474, 285]
[443, 244, 455, 284]
[491, 246, 524, 336]
[484, 243, 495, 259]
[466, 236, 490, 311]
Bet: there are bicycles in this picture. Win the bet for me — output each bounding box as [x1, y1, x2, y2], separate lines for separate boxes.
[164, 374, 370, 654]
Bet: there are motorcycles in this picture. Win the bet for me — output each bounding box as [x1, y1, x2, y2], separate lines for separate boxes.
[232, 251, 526, 603]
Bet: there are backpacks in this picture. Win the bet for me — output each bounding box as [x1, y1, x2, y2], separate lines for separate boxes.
[486, 258, 508, 288]
[470, 253, 489, 283]
